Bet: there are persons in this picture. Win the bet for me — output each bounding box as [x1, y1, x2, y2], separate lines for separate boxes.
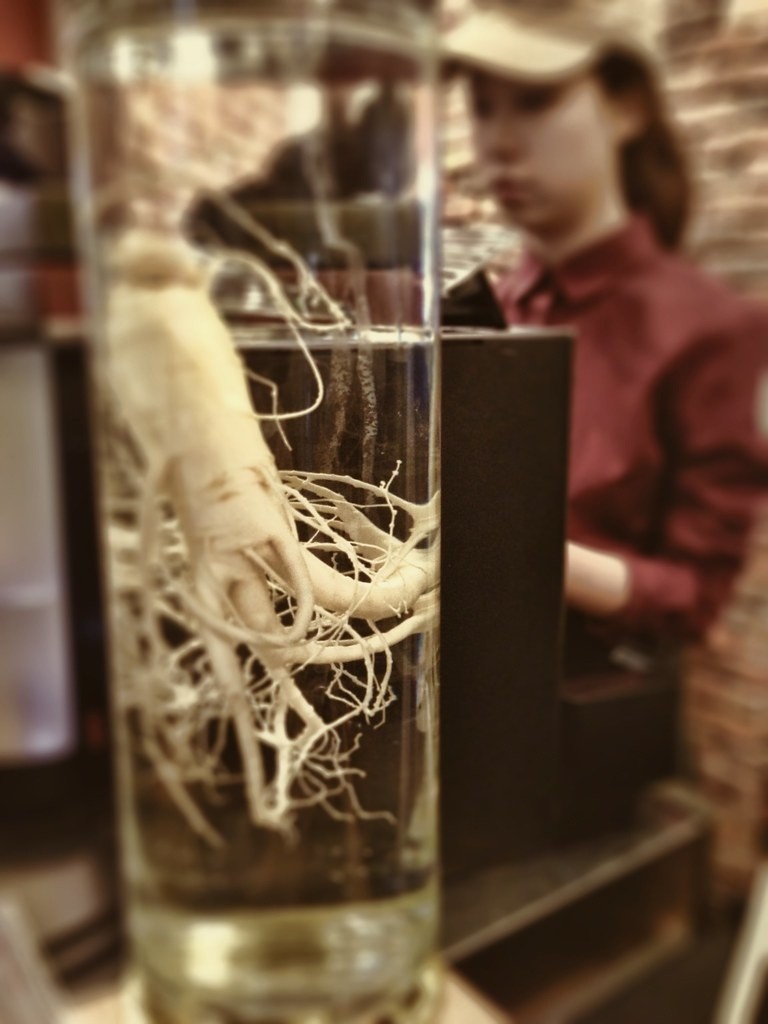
[435, 0, 768, 663]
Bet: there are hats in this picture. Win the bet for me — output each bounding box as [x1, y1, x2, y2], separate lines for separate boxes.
[436, 0, 666, 85]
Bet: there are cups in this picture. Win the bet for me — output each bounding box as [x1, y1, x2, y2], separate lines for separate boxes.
[54, 0, 445, 1024]
